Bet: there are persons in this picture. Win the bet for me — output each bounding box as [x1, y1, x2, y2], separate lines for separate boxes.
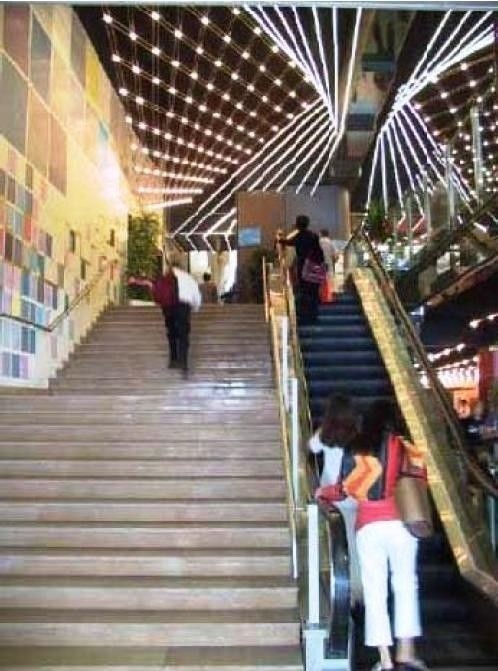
[457, 398, 471, 420]
[308, 396, 436, 671]
[195, 270, 219, 304]
[159, 249, 201, 373]
[278, 215, 340, 325]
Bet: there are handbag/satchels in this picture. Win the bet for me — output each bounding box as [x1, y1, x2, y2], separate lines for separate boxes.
[319, 274, 335, 303]
[301, 258, 326, 285]
[152, 266, 179, 311]
[391, 439, 435, 542]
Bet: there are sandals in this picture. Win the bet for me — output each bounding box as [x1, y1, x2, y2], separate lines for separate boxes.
[370, 657, 430, 671]
